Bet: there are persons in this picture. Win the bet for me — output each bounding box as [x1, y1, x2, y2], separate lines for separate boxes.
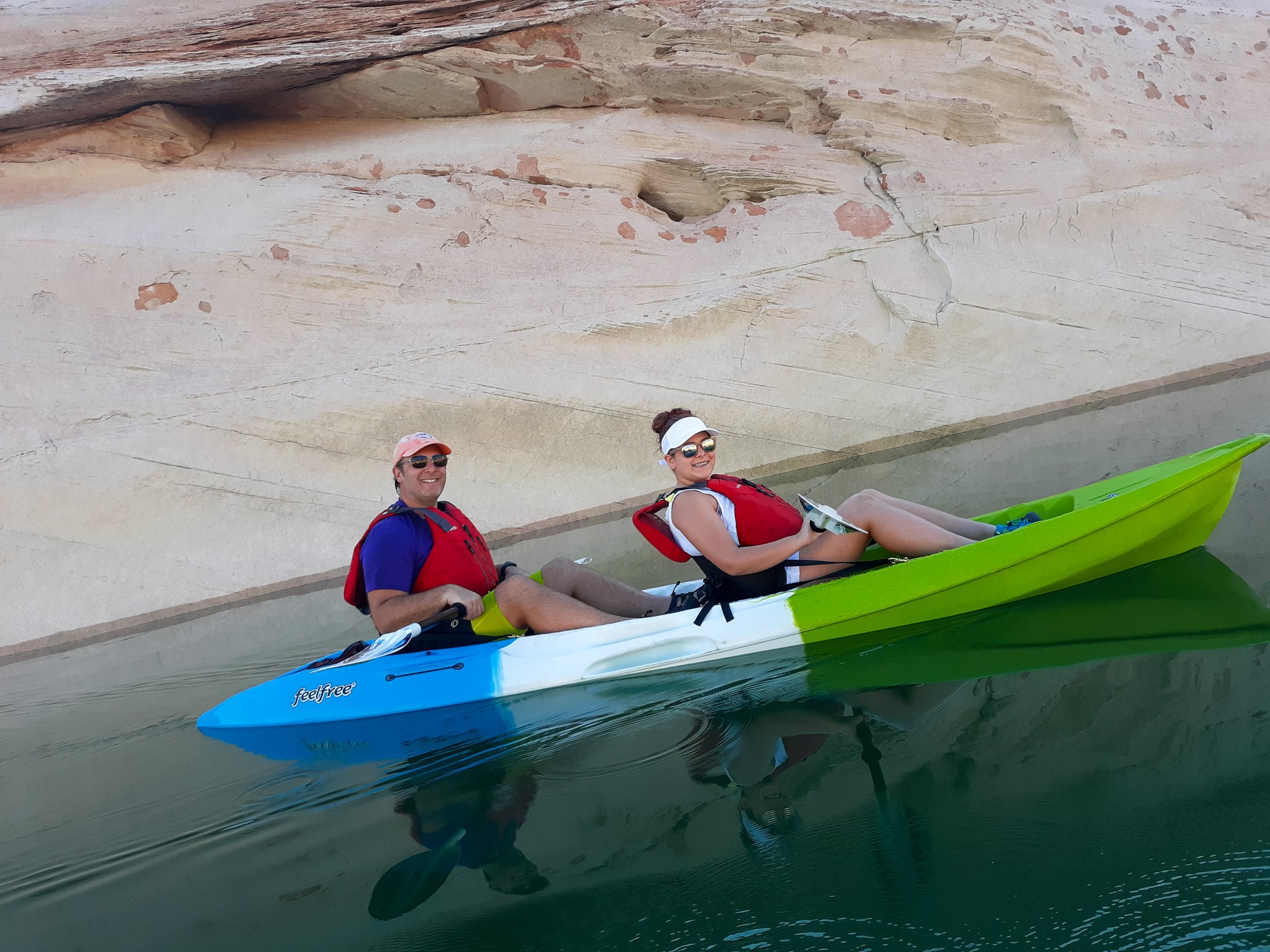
[357, 432, 709, 654]
[652, 407, 1039, 603]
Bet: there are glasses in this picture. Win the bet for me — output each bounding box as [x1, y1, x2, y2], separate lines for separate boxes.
[398, 455, 449, 469]
[669, 437, 718, 459]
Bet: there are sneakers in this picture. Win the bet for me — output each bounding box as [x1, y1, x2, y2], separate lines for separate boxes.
[995, 512, 1040, 536]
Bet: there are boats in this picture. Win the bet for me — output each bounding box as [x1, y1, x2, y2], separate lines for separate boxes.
[197, 427, 1270, 766]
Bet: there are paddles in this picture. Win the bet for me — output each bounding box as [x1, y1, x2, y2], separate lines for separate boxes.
[366, 757, 532, 921]
[853, 707, 913, 850]
[795, 491, 868, 536]
[304, 556, 593, 675]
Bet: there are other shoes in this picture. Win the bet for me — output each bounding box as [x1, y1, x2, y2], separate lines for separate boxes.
[668, 581, 707, 609]
[641, 597, 700, 617]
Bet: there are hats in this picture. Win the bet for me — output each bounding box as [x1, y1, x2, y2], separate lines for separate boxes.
[392, 432, 452, 466]
[658, 416, 719, 467]
